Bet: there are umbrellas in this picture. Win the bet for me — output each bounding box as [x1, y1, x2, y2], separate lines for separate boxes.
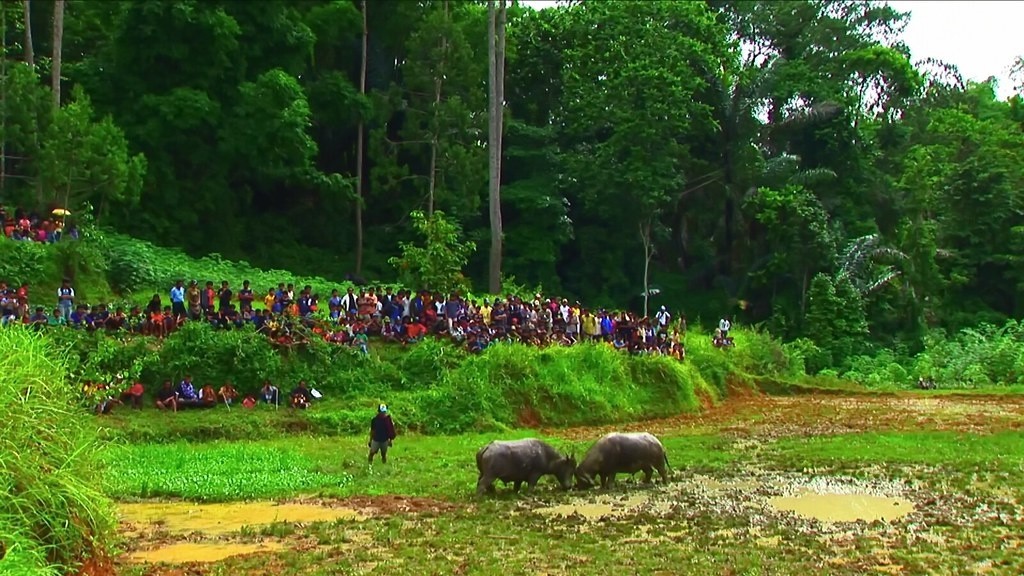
[52, 208, 72, 216]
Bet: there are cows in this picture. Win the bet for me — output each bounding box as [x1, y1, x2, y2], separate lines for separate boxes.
[474, 432, 577, 493]
[574, 431, 674, 489]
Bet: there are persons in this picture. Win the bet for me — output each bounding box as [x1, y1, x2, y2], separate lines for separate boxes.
[289, 380, 311, 408]
[175, 374, 198, 408]
[367, 405, 396, 464]
[155, 378, 180, 413]
[0, 204, 79, 246]
[81, 374, 144, 415]
[216, 379, 239, 404]
[0, 271, 686, 363]
[712, 316, 736, 351]
[243, 394, 256, 409]
[260, 379, 281, 405]
[196, 383, 216, 407]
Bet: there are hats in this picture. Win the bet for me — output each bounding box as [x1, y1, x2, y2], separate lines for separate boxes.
[546, 299, 551, 303]
[192, 279, 198, 285]
[661, 305, 666, 310]
[562, 298, 567, 303]
[576, 301, 581, 305]
[384, 316, 390, 323]
[533, 300, 539, 305]
[536, 294, 541, 297]
[378, 404, 387, 412]
[484, 298, 490, 304]
